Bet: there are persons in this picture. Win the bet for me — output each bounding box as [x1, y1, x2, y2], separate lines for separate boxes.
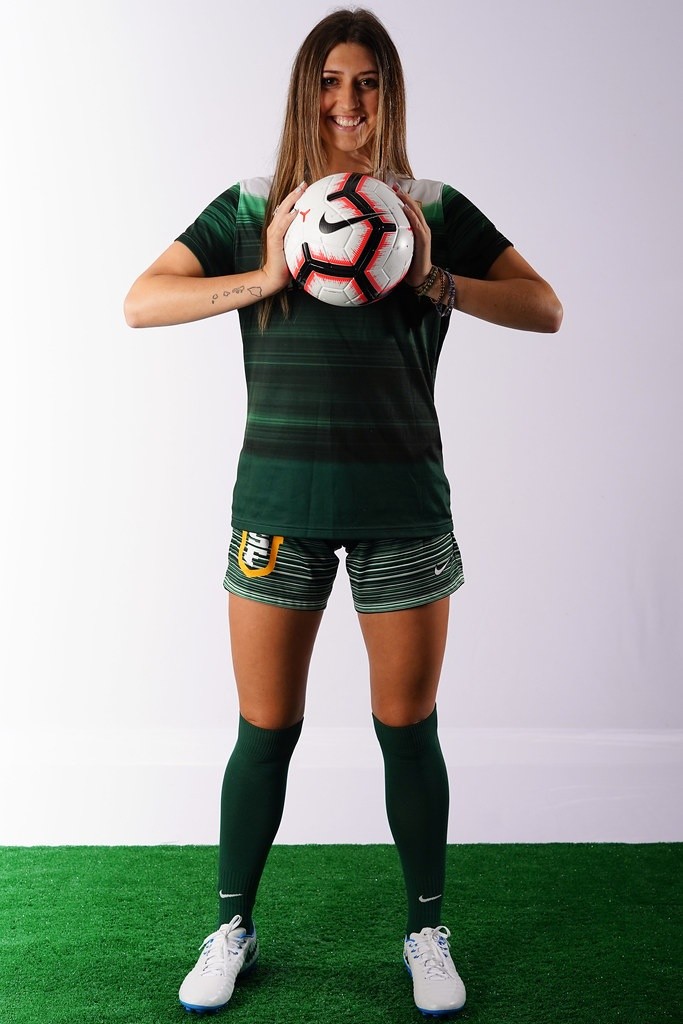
[124, 9, 564, 1012]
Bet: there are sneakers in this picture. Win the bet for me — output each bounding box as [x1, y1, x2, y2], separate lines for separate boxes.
[404, 928, 469, 1017]
[176, 922, 260, 1016]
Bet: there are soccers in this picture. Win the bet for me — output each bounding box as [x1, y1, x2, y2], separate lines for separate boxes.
[283, 171, 415, 306]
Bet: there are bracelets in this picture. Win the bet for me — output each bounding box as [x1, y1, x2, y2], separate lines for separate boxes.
[429, 267, 445, 304]
[434, 271, 456, 317]
[414, 265, 438, 296]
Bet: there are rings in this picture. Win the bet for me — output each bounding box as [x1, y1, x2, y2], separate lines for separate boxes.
[272, 204, 280, 217]
